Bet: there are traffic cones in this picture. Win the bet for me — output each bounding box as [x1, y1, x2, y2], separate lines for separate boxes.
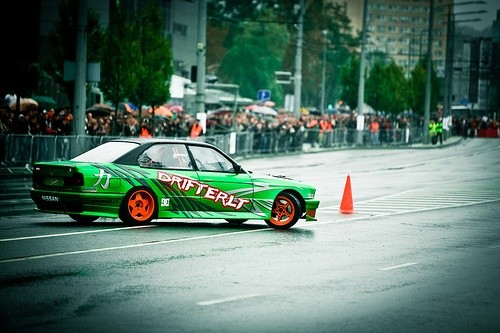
[339, 174, 353, 214]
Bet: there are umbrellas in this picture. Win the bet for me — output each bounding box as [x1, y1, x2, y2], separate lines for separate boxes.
[124, 104, 132, 113]
[86, 107, 110, 118]
[9, 98, 38, 111]
[149, 106, 173, 117]
[33, 96, 56, 105]
[324, 109, 337, 114]
[308, 109, 322, 116]
[128, 103, 137, 111]
[118, 104, 127, 112]
[253, 107, 278, 117]
[170, 106, 183, 114]
[263, 101, 275, 107]
[247, 105, 260, 110]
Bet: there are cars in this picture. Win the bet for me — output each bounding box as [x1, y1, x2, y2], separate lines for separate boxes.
[30, 139, 320, 229]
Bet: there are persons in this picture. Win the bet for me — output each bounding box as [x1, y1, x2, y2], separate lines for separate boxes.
[0, 94, 500, 166]
